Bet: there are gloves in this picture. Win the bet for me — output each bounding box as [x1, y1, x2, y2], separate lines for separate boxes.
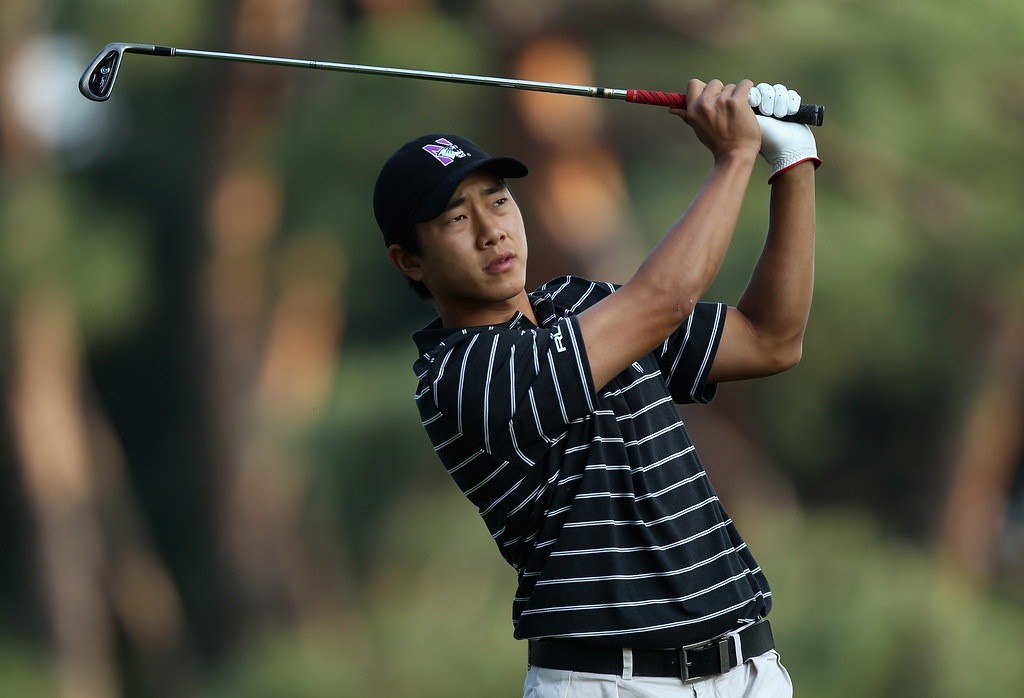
[748, 83, 822, 185]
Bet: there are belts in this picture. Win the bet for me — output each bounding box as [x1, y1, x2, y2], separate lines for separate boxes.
[529, 620, 775, 684]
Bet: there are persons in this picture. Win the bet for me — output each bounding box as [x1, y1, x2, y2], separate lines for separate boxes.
[371, 78, 824, 698]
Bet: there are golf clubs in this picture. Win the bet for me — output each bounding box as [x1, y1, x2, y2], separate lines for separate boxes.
[75, 40, 827, 128]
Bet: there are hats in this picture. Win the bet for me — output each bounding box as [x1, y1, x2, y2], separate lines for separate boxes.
[373, 133, 529, 239]
